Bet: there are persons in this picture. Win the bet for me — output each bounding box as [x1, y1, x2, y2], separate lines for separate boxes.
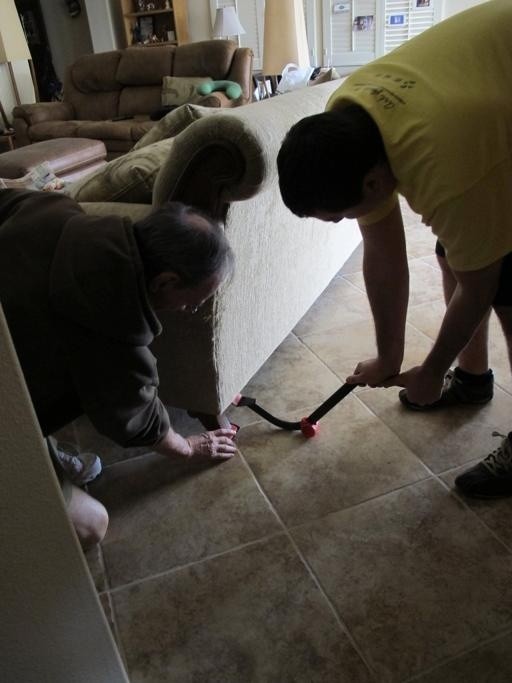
[277, 1, 512, 499]
[1, 188, 239, 554]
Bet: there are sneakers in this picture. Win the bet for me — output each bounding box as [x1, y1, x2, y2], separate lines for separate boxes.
[454, 430, 511, 499]
[59, 450, 102, 488]
[399, 364, 494, 412]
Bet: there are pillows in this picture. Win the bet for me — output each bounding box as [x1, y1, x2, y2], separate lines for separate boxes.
[63, 101, 221, 203]
[159, 74, 244, 109]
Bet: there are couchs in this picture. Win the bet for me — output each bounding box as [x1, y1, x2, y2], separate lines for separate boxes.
[12, 38, 255, 159]
[0, 77, 374, 443]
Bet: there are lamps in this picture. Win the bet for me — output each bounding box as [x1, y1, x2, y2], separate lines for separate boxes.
[209, 6, 247, 49]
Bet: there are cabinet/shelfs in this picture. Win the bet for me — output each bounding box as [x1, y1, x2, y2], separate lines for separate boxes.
[119, 0, 190, 47]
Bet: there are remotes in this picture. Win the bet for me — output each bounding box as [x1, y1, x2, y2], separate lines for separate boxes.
[111, 115, 134, 121]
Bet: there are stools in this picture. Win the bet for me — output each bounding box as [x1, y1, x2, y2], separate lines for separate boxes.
[1, 136, 110, 200]
